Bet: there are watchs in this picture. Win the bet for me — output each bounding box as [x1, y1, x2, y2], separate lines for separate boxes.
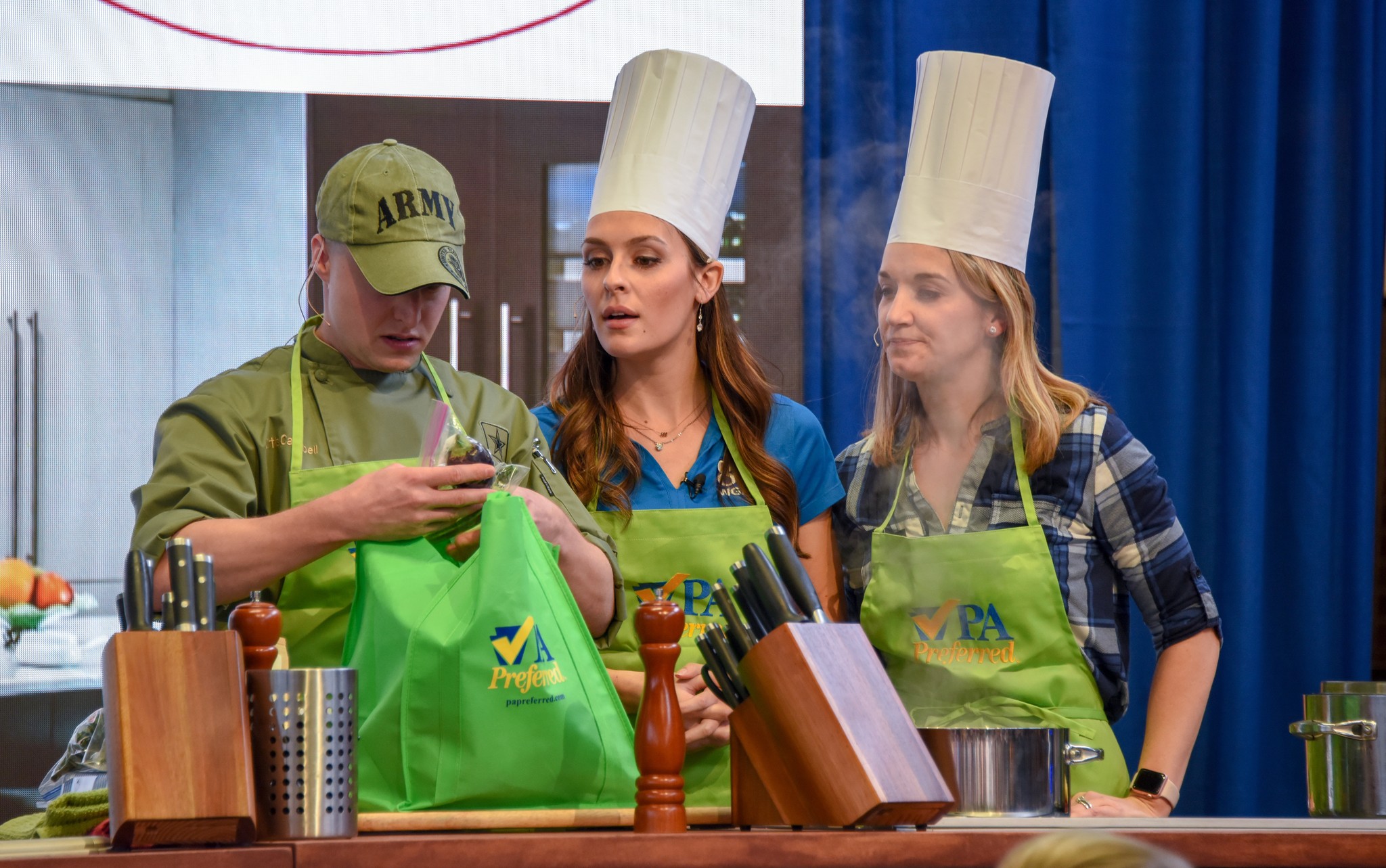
[1127, 768, 1179, 811]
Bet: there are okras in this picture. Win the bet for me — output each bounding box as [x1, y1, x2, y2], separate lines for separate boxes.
[427, 465, 519, 542]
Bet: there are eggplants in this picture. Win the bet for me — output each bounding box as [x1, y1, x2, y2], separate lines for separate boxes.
[446, 434, 497, 490]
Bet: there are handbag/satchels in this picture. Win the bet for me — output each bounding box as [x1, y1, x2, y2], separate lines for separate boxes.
[343, 493, 642, 811]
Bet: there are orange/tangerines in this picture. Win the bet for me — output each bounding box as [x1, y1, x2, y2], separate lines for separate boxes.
[0, 557, 35, 609]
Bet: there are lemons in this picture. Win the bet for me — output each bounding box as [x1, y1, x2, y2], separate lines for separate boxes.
[7, 604, 46, 631]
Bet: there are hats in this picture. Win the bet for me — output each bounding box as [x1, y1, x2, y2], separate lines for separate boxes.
[885, 51, 1056, 274]
[587, 48, 757, 260]
[314, 138, 471, 300]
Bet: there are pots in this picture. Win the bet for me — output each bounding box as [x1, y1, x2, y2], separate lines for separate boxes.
[918, 725, 1104, 819]
[1290, 694, 1386, 819]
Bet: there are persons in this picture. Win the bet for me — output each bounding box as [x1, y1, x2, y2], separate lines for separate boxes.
[128, 139, 627, 670]
[831, 193, 1224, 819]
[525, 168, 850, 808]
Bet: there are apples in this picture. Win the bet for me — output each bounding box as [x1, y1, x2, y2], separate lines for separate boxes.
[36, 572, 74, 610]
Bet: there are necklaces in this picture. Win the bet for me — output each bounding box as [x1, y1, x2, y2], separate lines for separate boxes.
[619, 400, 711, 452]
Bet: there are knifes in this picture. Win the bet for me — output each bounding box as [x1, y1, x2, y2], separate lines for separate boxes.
[117, 539, 217, 636]
[698, 525, 833, 708]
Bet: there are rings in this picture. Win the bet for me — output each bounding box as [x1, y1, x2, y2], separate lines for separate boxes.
[1077, 795, 1092, 811]
